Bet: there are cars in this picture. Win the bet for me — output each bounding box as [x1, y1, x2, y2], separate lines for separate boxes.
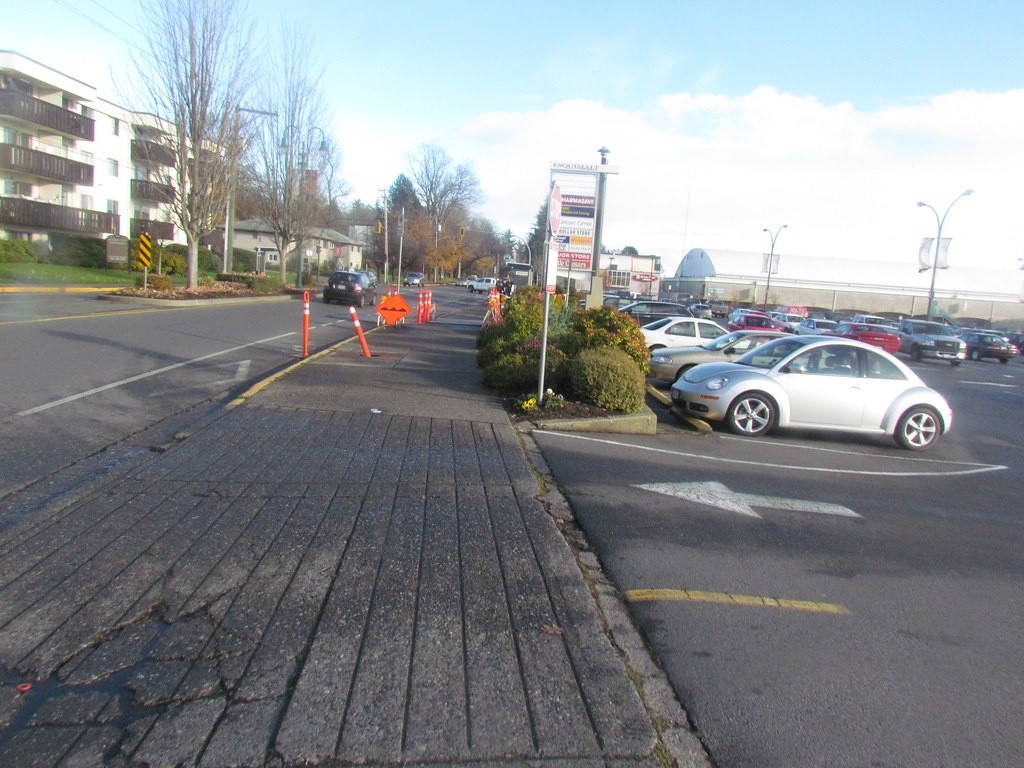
[578, 293, 1024, 362]
[820, 322, 901, 355]
[727, 314, 782, 343]
[638, 317, 763, 352]
[456, 275, 477, 287]
[356, 271, 377, 288]
[793, 318, 838, 336]
[645, 330, 852, 386]
[955, 332, 1019, 364]
[323, 271, 377, 308]
[403, 272, 424, 288]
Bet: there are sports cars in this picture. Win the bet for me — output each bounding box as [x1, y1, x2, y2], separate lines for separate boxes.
[671, 335, 953, 452]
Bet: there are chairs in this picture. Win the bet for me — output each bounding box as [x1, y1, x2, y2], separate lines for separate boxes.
[821, 353, 855, 376]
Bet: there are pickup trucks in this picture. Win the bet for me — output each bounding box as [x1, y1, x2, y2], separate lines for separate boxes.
[883, 317, 967, 366]
[466, 278, 516, 294]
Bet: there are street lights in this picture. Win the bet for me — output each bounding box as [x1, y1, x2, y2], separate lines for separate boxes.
[276, 125, 330, 288]
[762, 224, 789, 312]
[916, 189, 974, 322]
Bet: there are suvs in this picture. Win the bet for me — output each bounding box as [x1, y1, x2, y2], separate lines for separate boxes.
[616, 301, 694, 327]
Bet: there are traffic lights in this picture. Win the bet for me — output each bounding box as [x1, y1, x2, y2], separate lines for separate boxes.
[459, 227, 465, 237]
